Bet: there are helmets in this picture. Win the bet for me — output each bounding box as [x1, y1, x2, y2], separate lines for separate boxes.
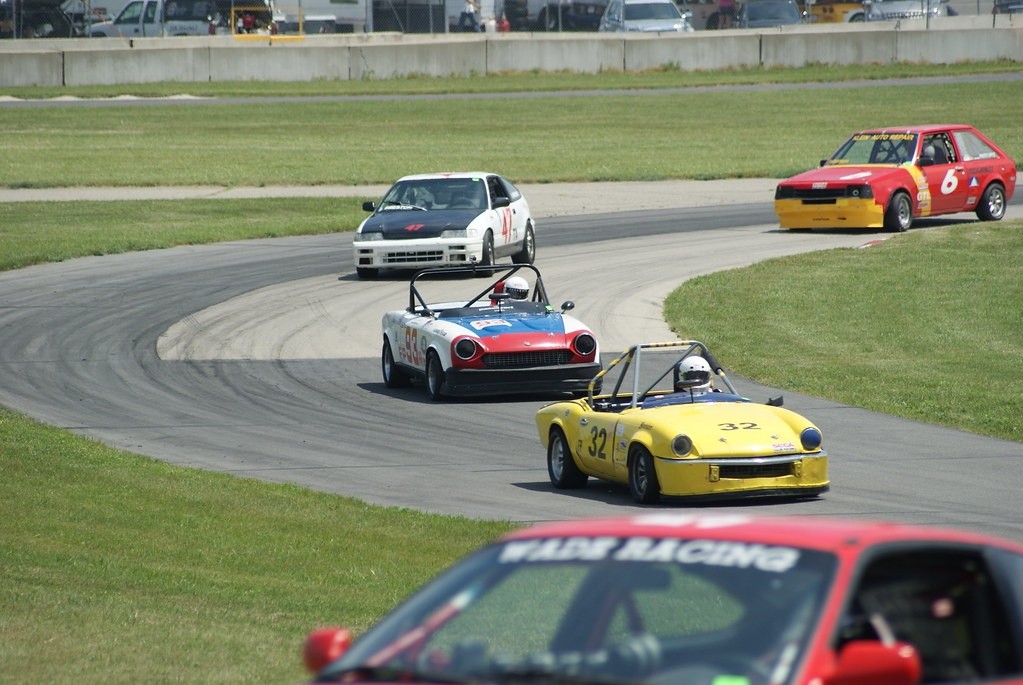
[678, 356, 712, 392]
[503, 276, 529, 303]
[920, 144, 935, 158]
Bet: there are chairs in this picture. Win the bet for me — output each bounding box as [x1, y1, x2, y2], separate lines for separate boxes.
[674, 362, 686, 393]
[864, 574, 995, 685]
[491, 282, 505, 304]
[933, 144, 948, 163]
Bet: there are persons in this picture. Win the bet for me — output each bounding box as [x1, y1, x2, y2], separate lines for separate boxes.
[457, 0, 482, 32]
[717, 0, 738, 30]
[503, 277, 531, 305]
[678, 355, 712, 394]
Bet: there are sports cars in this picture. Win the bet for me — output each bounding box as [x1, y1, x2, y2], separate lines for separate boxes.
[378, 264, 602, 399]
[534, 338, 831, 507]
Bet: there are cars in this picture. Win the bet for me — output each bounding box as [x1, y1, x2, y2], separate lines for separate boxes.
[353, 172, 536, 285]
[774, 125, 1017, 234]
[305, 512, 1022, 685]
[0, 0, 1021, 42]
